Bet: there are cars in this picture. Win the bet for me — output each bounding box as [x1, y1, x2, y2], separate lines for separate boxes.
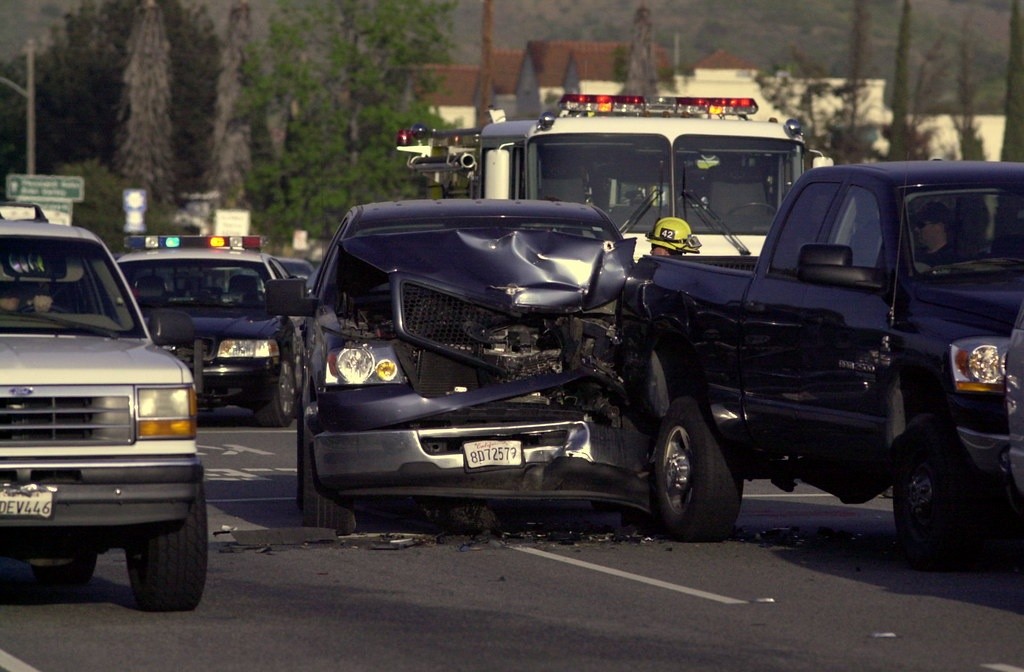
[268, 198, 660, 523]
[115, 234, 305, 425]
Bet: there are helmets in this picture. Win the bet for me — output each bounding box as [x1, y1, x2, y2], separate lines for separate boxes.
[645, 217, 701, 254]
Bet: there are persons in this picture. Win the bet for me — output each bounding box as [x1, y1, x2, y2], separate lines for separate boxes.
[915, 204, 971, 263]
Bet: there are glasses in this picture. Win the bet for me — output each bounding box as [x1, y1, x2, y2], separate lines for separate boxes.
[914, 222, 932, 228]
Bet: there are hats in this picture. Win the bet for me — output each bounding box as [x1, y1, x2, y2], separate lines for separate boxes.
[915, 202, 949, 223]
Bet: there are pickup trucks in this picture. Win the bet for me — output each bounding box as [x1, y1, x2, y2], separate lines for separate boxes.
[620, 160, 1023, 556]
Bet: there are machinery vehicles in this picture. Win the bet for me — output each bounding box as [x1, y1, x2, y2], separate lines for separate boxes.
[397, 90, 835, 271]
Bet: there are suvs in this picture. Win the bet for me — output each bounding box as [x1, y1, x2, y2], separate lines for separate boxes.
[0, 220, 208, 613]
